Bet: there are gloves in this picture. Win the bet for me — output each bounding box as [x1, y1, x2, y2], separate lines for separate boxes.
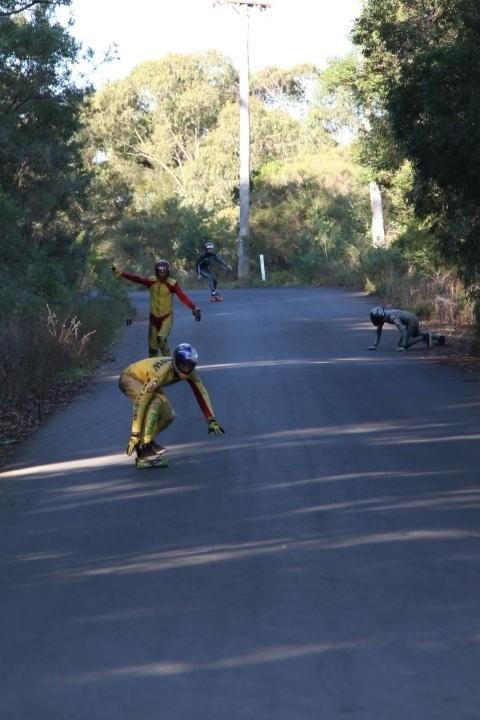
[127, 434, 140, 456]
[366, 345, 375, 350]
[193, 308, 201, 322]
[207, 420, 226, 438]
[197, 275, 203, 280]
[111, 264, 122, 276]
[396, 347, 407, 352]
[227, 265, 232, 271]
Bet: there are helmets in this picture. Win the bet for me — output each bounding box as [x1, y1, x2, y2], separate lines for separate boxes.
[370, 306, 385, 326]
[205, 243, 213, 249]
[173, 343, 198, 380]
[155, 260, 170, 270]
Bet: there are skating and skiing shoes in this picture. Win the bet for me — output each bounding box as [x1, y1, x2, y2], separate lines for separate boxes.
[210, 295, 224, 302]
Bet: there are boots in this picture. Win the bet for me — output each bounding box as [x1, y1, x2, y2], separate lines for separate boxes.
[135, 442, 168, 469]
[424, 332, 444, 349]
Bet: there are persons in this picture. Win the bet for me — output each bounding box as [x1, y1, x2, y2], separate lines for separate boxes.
[119, 343, 225, 469]
[196, 243, 232, 302]
[365, 307, 445, 352]
[111, 259, 201, 358]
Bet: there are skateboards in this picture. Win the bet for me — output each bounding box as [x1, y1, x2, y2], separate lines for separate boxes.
[135, 453, 169, 470]
[211, 293, 223, 301]
